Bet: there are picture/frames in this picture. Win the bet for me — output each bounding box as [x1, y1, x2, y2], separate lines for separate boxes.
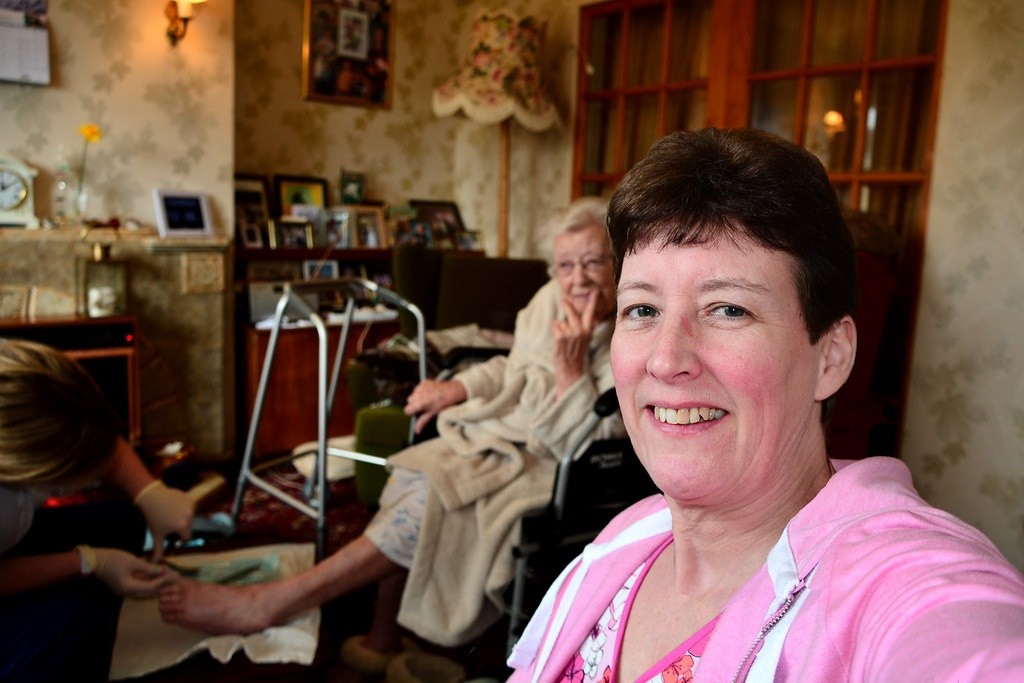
[302, 259, 342, 279]
[236, 172, 273, 232]
[349, 207, 387, 249]
[409, 198, 466, 247]
[238, 246, 399, 455]
[273, 172, 332, 224]
[302, 0, 399, 111]
[153, 188, 216, 239]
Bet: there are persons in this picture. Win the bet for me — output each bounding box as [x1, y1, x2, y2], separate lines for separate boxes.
[506, 128, 1024, 683]
[428, 212, 456, 245]
[0, 338, 196, 601]
[159, 195, 627, 646]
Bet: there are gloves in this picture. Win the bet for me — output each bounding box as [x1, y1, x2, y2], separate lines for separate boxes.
[133, 479, 196, 562]
[73, 544, 169, 597]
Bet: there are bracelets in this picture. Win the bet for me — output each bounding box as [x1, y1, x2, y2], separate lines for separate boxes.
[76, 544, 96, 570]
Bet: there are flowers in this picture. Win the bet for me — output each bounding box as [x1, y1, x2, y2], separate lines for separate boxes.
[56, 123, 105, 175]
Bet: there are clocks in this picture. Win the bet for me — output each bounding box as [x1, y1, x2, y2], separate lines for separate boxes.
[0, 158, 39, 230]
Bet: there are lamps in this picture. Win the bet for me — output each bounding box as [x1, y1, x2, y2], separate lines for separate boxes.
[430, 6, 564, 258]
[165, 0, 207, 46]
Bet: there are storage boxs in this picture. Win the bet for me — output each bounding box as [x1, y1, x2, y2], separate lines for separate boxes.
[247, 259, 300, 281]
[250, 282, 318, 322]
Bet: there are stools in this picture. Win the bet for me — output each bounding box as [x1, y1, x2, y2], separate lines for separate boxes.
[72, 525, 340, 682]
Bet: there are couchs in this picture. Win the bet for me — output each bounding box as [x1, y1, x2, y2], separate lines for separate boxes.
[347, 356, 410, 512]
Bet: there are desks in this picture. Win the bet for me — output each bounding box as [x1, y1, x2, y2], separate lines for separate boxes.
[408, 252, 555, 370]
[0, 309, 136, 448]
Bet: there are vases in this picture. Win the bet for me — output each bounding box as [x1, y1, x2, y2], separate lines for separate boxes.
[71, 179, 90, 222]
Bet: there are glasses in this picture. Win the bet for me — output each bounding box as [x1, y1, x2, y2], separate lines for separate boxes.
[547, 254, 615, 279]
